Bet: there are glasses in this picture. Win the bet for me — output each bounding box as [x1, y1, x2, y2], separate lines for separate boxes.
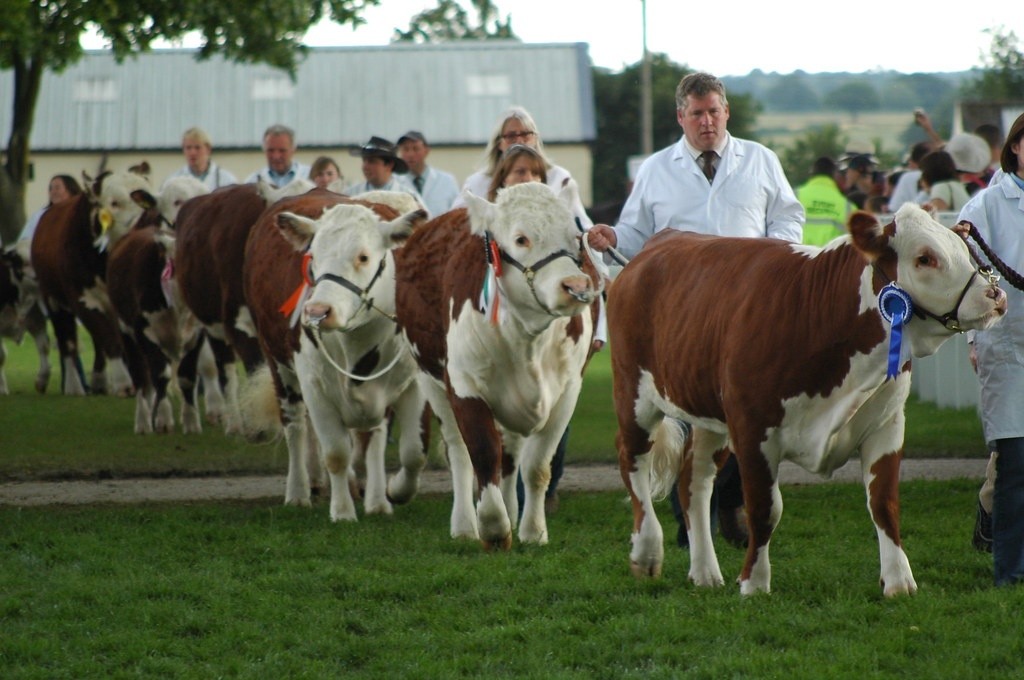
[499, 130, 534, 145]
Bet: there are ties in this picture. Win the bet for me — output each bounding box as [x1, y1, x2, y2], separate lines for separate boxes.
[699, 150, 720, 185]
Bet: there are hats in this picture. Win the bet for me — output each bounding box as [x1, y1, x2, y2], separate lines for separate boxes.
[349, 136, 410, 176]
[397, 130, 428, 146]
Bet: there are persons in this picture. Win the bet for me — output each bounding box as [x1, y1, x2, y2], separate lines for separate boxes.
[587, 72, 806, 549]
[16, 175, 89, 393]
[163, 128, 239, 193]
[245, 123, 310, 187]
[462, 106, 585, 211]
[308, 156, 346, 188]
[346, 136, 433, 221]
[949, 114, 1024, 588]
[794, 108, 1005, 248]
[487, 145, 606, 517]
[398, 131, 460, 219]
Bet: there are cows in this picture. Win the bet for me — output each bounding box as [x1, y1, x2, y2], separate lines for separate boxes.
[0, 161, 152, 394]
[607, 204, 1007, 597]
[395, 179, 597, 549]
[244, 190, 426, 523]
[175, 185, 272, 434]
[103, 227, 204, 435]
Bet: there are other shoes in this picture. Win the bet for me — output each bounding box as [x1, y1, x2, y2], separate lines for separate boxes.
[542, 488, 560, 515]
[970, 501, 993, 553]
[678, 519, 693, 548]
